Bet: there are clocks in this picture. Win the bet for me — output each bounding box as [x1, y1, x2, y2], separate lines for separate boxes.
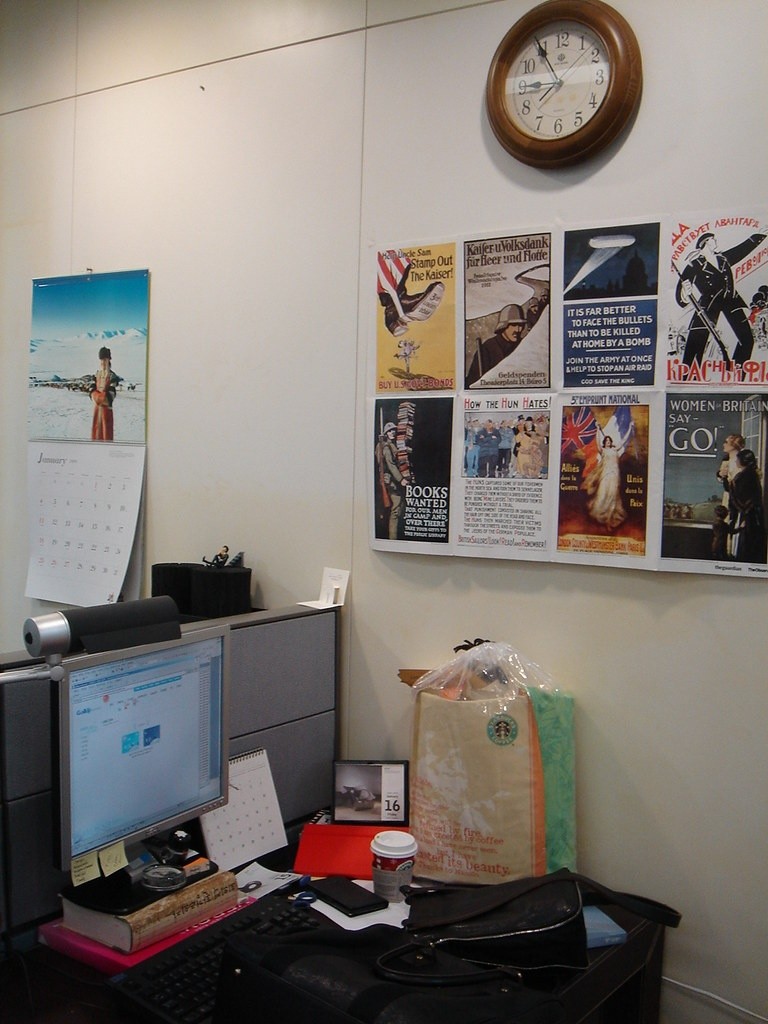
[485, 0, 643, 170]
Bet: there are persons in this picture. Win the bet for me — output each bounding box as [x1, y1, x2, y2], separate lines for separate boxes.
[89, 347, 119, 441]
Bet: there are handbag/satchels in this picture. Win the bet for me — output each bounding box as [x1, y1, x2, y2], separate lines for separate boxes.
[400, 868, 682, 972]
[212, 921, 570, 1024]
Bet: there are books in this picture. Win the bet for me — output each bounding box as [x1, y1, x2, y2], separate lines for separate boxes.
[57, 865, 239, 955]
[583, 905, 629, 946]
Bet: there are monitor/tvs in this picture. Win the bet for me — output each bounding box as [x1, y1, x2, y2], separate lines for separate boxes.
[56, 621, 229, 916]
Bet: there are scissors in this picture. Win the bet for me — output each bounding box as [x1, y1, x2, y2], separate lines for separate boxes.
[287, 891, 317, 911]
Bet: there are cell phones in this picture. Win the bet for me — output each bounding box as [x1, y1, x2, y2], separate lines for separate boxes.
[302, 876, 389, 917]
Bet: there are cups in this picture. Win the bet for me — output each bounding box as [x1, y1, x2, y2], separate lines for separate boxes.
[369, 831, 419, 903]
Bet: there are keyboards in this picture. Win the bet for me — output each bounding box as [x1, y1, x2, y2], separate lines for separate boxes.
[105, 896, 335, 1024]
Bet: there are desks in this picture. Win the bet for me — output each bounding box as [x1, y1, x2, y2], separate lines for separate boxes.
[0, 829, 661, 1024]
